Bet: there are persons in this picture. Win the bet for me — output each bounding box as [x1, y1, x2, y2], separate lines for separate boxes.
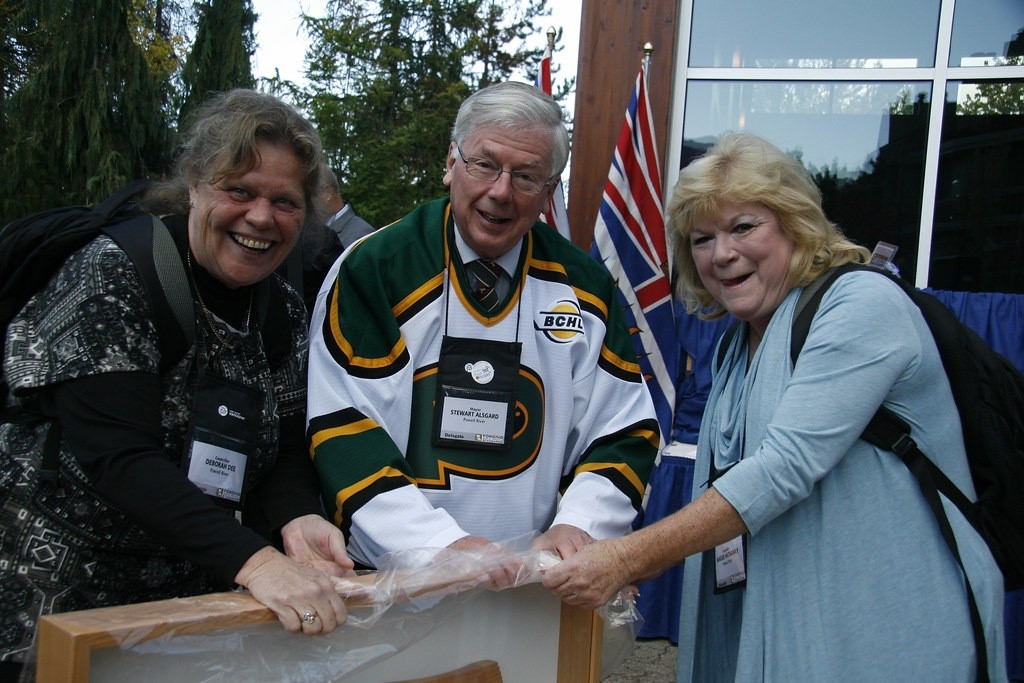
[541, 131, 1008, 683]
[314, 163, 376, 250]
[2, 86, 364, 682]
[305, 78, 666, 592]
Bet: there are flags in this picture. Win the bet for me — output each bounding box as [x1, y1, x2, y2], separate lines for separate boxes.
[591, 60, 686, 442]
[534, 48, 572, 242]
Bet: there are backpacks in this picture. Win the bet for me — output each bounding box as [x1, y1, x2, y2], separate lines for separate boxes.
[717, 262, 1024, 592]
[0, 180, 289, 429]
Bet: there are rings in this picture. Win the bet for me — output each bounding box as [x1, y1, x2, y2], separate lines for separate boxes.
[301, 612, 317, 624]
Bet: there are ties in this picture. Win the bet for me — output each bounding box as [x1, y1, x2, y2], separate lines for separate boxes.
[468, 258, 502, 314]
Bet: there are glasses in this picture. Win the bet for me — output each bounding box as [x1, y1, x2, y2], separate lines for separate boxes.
[454, 139, 560, 197]
